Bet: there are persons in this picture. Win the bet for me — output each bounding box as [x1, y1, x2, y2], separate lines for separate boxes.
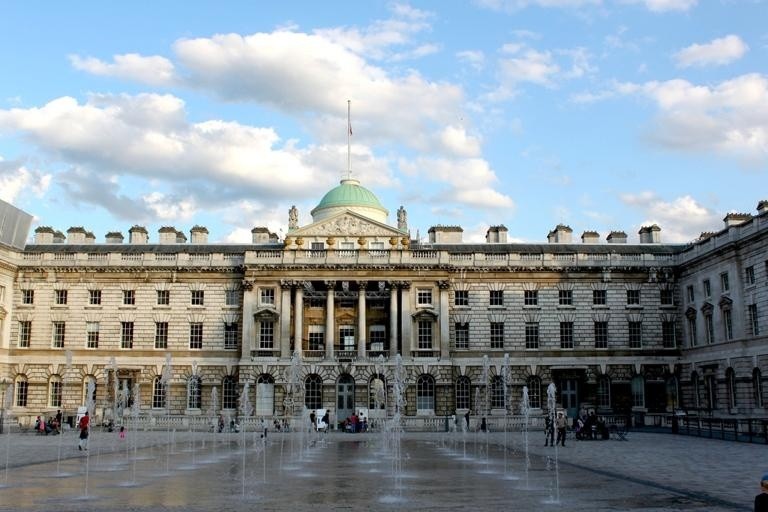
[463, 410, 470, 430]
[214, 405, 374, 441]
[480, 417, 487, 432]
[754, 473, 768, 512]
[542, 408, 608, 448]
[31, 408, 127, 442]
[77, 411, 90, 451]
